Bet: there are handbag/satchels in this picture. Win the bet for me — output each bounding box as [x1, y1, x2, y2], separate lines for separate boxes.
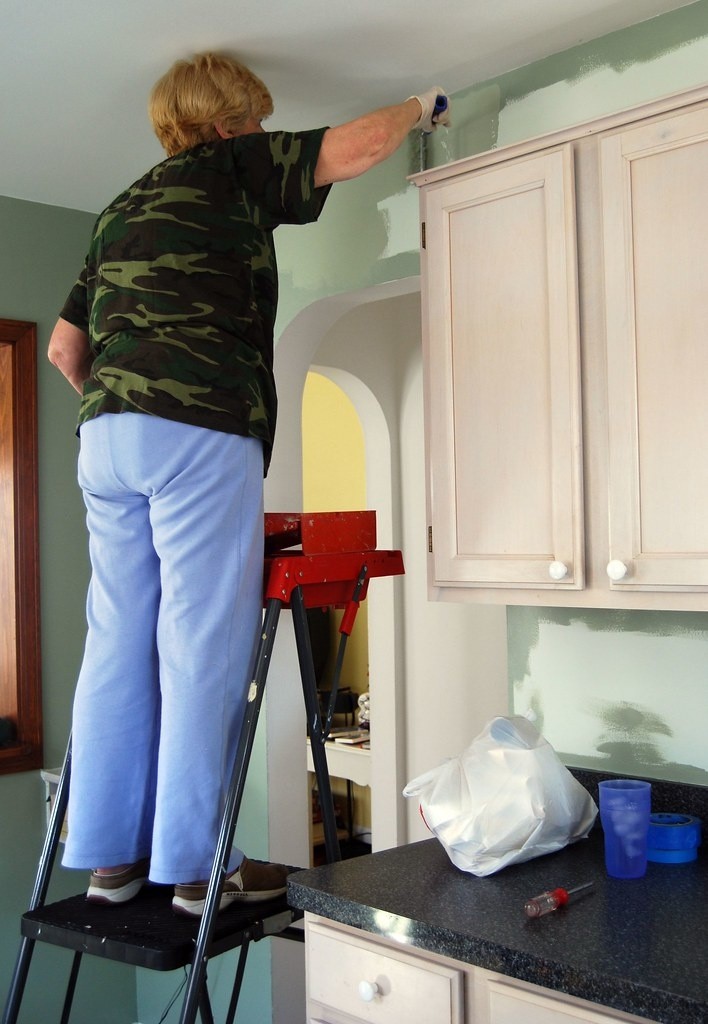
[402, 715, 599, 877]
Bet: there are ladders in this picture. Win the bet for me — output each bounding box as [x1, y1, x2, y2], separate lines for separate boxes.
[0, 511, 408, 1024]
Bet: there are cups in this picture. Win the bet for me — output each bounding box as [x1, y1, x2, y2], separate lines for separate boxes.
[599, 779, 651, 880]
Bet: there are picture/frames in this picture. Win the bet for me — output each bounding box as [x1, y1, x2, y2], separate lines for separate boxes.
[0, 316, 44, 776]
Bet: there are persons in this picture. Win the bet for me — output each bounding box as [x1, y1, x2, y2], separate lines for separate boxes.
[47, 54, 453, 914]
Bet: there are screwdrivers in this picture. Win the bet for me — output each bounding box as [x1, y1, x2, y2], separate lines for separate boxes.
[525, 880, 595, 917]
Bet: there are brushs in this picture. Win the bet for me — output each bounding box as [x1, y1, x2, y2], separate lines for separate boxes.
[410, 96, 447, 181]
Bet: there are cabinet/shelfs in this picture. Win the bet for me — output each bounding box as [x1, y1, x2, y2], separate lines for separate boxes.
[294, 911, 656, 1024]
[411, 86, 708, 616]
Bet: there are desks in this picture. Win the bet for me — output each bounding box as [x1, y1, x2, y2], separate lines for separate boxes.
[305, 735, 372, 789]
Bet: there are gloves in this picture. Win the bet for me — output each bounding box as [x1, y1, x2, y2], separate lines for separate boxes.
[405, 87, 452, 132]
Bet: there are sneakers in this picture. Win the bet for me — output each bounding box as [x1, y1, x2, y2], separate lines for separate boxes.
[86, 856, 151, 903]
[172, 854, 294, 916]
